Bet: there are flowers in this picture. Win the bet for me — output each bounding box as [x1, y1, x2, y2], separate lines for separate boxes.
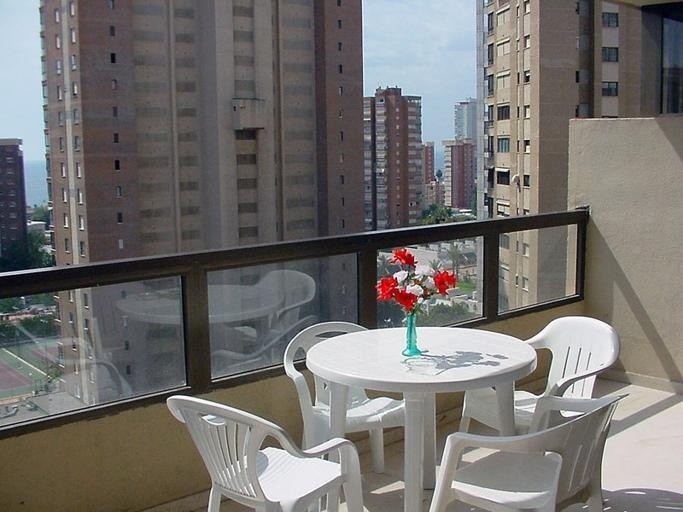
[374, 246, 461, 319]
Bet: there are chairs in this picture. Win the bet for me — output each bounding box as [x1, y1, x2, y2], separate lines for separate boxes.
[453, 313, 621, 471]
[11, 318, 125, 396]
[210, 316, 312, 374]
[284, 320, 405, 475]
[166, 395, 366, 510]
[426, 391, 629, 509]
[226, 269, 317, 352]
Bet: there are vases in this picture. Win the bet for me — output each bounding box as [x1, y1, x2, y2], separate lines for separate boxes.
[403, 314, 421, 358]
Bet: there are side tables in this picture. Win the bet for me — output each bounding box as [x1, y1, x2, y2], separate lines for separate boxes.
[116, 284, 286, 396]
[305, 326, 538, 511]
